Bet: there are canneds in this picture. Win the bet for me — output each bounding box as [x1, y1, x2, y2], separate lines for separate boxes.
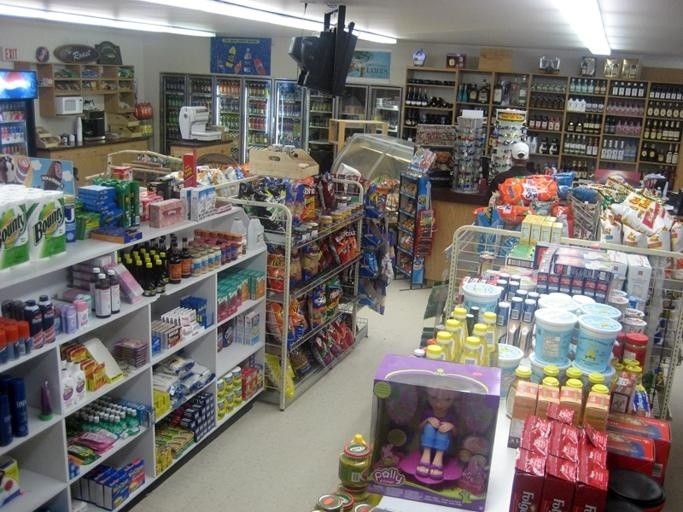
[510, 275, 521, 283]
[499, 273, 510, 283]
[515, 289, 528, 300]
[528, 292, 539, 300]
[185, 244, 221, 277]
[522, 299, 537, 323]
[496, 302, 510, 327]
[506, 281, 520, 302]
[509, 296, 523, 321]
[497, 279, 507, 302]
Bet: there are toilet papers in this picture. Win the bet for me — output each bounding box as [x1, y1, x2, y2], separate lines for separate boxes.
[0, 182, 69, 271]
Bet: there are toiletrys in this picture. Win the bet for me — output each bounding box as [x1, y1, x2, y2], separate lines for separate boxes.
[40, 378, 54, 421]
[75, 293, 92, 321]
[72, 299, 88, 329]
[0, 385, 15, 447]
[7, 377, 29, 438]
[54, 304, 76, 334]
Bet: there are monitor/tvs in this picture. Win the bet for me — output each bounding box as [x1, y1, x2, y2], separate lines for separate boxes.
[287, 26, 357, 95]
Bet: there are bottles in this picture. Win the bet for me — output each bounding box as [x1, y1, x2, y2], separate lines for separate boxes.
[611, 81, 617, 95]
[24, 299, 44, 349]
[594, 113, 600, 134]
[579, 136, 586, 154]
[600, 138, 606, 159]
[672, 102, 678, 118]
[606, 139, 612, 159]
[89, 267, 102, 311]
[603, 116, 609, 133]
[629, 83, 637, 97]
[600, 80, 605, 95]
[582, 114, 588, 133]
[616, 82, 624, 96]
[670, 143, 678, 164]
[37, 294, 56, 342]
[649, 85, 682, 100]
[569, 135, 575, 153]
[665, 143, 672, 164]
[246, 81, 270, 145]
[586, 136, 592, 156]
[587, 115, 593, 133]
[623, 82, 630, 96]
[617, 140, 624, 161]
[121, 231, 192, 296]
[569, 78, 575, 92]
[563, 133, 569, 153]
[647, 143, 656, 161]
[416, 87, 421, 106]
[665, 102, 672, 118]
[659, 102, 665, 118]
[652, 102, 659, 116]
[107, 269, 121, 313]
[425, 306, 499, 370]
[673, 122, 680, 142]
[405, 86, 410, 105]
[421, 88, 428, 107]
[94, 273, 111, 318]
[656, 121, 662, 140]
[678, 103, 682, 119]
[587, 79, 593, 93]
[216, 76, 240, 131]
[164, 78, 185, 140]
[575, 78, 580, 93]
[650, 119, 656, 140]
[410, 87, 416, 105]
[592, 138, 598, 156]
[646, 100, 652, 116]
[668, 121, 674, 141]
[405, 109, 419, 125]
[594, 80, 599, 94]
[190, 77, 212, 124]
[661, 120, 669, 140]
[612, 140, 617, 161]
[657, 148, 664, 162]
[643, 120, 649, 139]
[608, 117, 615, 133]
[458, 83, 468, 102]
[581, 79, 587, 93]
[640, 142, 647, 161]
[574, 135, 580, 155]
[636, 83, 644, 97]
[503, 357, 611, 424]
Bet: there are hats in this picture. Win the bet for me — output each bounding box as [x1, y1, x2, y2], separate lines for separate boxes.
[512, 141, 530, 160]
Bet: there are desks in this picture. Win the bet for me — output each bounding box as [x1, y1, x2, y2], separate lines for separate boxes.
[169, 138, 233, 170]
[35, 133, 153, 181]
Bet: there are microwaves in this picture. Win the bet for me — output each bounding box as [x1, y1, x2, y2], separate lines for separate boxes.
[54, 96, 85, 116]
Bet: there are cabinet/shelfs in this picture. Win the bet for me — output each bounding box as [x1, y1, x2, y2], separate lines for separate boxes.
[393, 169, 436, 292]
[332, 133, 421, 191]
[403, 64, 683, 192]
[0, 206, 265, 512]
[162, 73, 273, 157]
[13, 60, 137, 119]
[275, 83, 403, 167]
[196, 174, 368, 411]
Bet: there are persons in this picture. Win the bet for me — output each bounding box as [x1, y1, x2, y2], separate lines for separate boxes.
[482, 142, 533, 201]
[378, 366, 490, 495]
